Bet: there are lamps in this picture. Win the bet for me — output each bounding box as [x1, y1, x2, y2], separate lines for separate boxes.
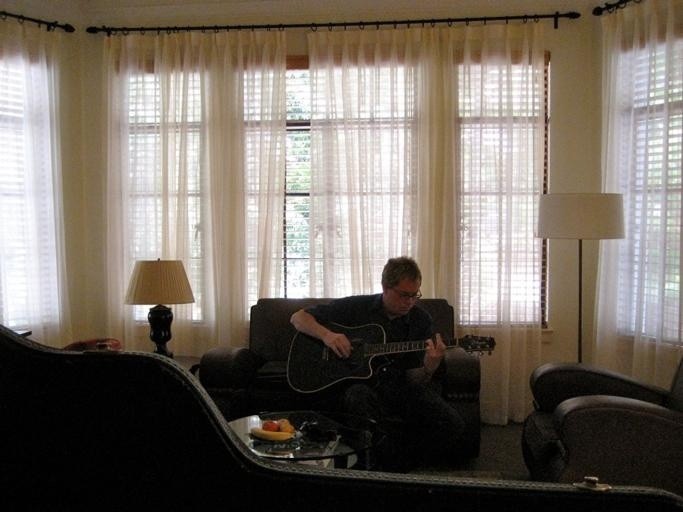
[122, 259, 195, 358]
[533, 192, 625, 367]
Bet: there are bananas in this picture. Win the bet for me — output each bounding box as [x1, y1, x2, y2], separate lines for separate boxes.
[251, 427, 295, 442]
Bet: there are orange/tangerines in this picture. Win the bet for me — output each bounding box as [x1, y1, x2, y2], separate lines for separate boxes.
[281, 423, 295, 434]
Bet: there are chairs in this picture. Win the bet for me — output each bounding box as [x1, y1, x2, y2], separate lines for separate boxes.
[521, 358, 683, 494]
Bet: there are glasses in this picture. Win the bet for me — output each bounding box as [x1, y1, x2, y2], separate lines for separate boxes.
[390, 285, 422, 300]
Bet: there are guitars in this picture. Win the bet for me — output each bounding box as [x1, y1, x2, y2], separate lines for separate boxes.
[287, 318, 496, 393]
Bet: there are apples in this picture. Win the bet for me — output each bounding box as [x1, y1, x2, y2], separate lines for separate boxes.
[263, 421, 280, 432]
[278, 419, 289, 427]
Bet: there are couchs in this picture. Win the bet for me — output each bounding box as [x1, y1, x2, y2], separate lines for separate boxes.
[199, 298, 481, 459]
[0, 328, 681, 510]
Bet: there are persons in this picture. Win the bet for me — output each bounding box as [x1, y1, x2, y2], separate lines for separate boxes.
[288, 257, 468, 477]
